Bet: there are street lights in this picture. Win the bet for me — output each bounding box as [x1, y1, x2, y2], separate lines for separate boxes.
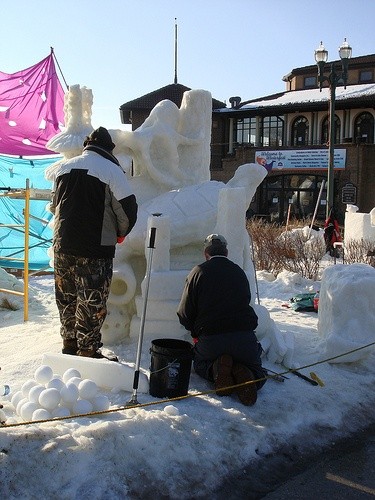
[314, 37, 353, 223]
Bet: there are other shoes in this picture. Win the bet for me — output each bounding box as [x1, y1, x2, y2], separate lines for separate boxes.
[75, 350, 118, 362]
[62, 345, 78, 355]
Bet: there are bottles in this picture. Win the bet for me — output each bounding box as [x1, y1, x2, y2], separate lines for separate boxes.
[313, 291, 319, 313]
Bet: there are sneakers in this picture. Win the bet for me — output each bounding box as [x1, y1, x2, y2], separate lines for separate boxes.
[212, 355, 257, 406]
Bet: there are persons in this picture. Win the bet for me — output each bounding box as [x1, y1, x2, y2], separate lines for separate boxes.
[176, 234, 268, 406]
[50, 126, 138, 361]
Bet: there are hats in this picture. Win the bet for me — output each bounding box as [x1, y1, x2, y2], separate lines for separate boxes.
[205, 233, 228, 250]
[83, 126, 116, 150]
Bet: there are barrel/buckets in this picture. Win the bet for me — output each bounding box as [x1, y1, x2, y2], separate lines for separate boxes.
[148, 335, 195, 400]
